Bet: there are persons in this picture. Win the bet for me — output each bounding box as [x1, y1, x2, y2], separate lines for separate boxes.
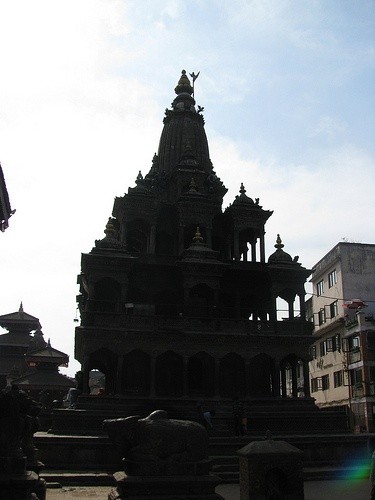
[195, 394, 274, 441]
